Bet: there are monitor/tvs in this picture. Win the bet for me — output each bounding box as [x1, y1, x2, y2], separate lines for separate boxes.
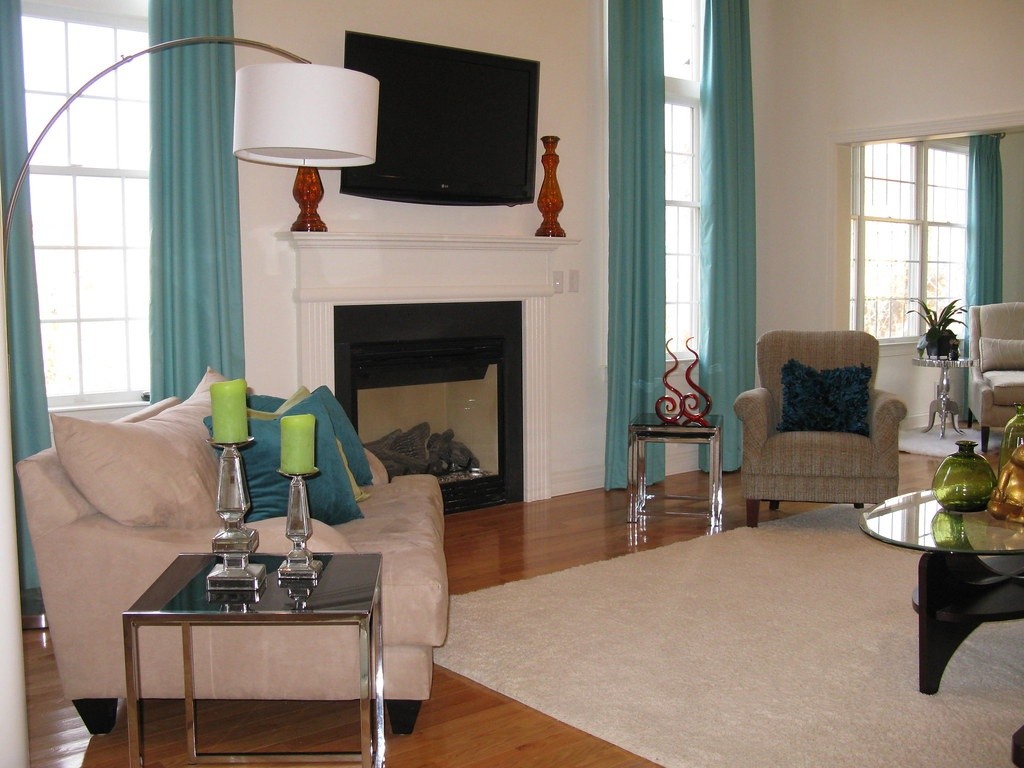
[338, 31, 540, 207]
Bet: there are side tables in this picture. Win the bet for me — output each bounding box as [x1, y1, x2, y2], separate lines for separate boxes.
[911, 358, 974, 440]
[628, 413, 724, 526]
[122, 552, 386, 767]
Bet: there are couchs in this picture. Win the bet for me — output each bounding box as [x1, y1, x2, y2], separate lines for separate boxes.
[732, 329, 907, 529]
[14, 398, 448, 737]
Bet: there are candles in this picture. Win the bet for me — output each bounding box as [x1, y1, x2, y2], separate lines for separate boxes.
[280, 413, 316, 473]
[210, 378, 250, 442]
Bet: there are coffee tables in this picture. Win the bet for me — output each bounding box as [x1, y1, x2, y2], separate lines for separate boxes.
[857, 490, 1024, 695]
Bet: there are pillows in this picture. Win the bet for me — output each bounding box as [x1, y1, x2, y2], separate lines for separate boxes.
[775, 359, 872, 439]
[46, 365, 227, 528]
[978, 337, 1024, 373]
[202, 383, 373, 527]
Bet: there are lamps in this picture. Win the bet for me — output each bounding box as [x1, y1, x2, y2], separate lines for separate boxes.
[5, 36, 380, 263]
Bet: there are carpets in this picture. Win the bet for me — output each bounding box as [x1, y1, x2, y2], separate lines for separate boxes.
[433, 504, 1024, 768]
[898, 426, 1002, 458]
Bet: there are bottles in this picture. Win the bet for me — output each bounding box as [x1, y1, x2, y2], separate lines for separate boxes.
[998, 402, 1024, 477]
[535, 136, 567, 237]
[931, 440, 996, 511]
[932, 508, 973, 550]
[949, 335, 960, 361]
[291, 167, 328, 232]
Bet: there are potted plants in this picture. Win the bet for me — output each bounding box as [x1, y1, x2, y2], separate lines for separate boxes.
[905, 297, 970, 360]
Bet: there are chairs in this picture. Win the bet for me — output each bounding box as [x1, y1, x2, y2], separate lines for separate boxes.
[967, 302, 1024, 453]
